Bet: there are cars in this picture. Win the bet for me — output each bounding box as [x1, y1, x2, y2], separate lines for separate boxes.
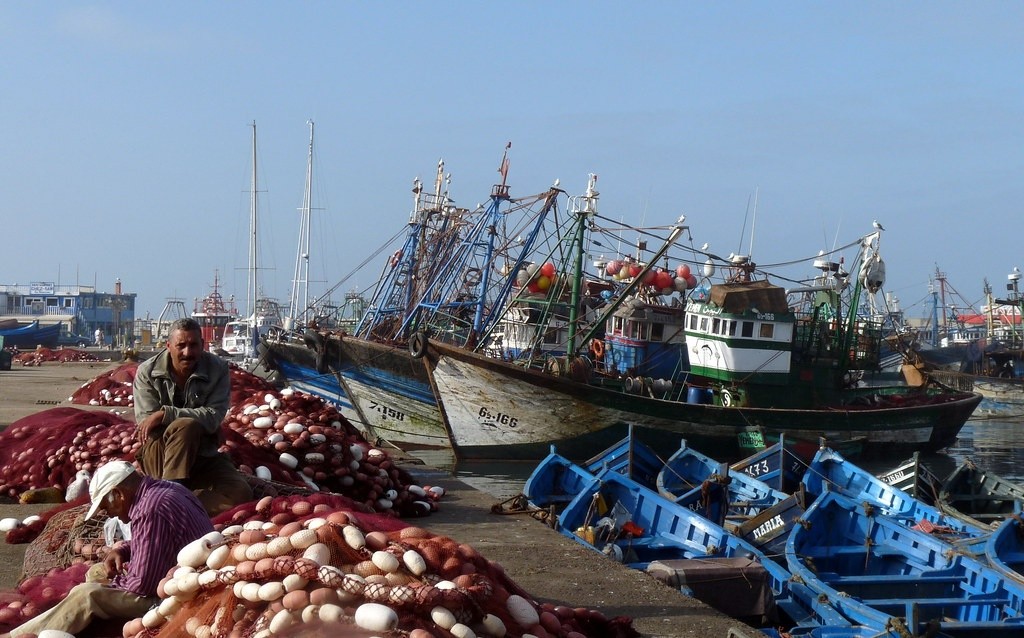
[57, 331, 93, 348]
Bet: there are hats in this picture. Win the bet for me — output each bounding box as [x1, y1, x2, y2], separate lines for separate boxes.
[84, 461, 135, 521]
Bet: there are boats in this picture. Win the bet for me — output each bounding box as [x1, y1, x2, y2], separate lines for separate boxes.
[0, 319, 61, 349]
[520, 426, 1024, 638]
[190, 117, 1024, 479]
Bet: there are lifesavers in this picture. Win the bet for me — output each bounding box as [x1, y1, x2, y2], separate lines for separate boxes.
[304, 330, 330, 374]
[865, 277, 877, 288]
[408, 332, 428, 358]
[999, 371, 1012, 379]
[464, 267, 483, 285]
[592, 339, 604, 358]
[255, 343, 277, 369]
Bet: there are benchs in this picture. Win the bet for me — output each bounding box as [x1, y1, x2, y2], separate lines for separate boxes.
[595, 538, 682, 552]
[862, 597, 1009, 636]
[821, 571, 968, 588]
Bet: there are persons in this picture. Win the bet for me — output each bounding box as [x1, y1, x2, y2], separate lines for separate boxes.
[131, 317, 234, 489]
[0, 461, 217, 637]
[92, 327, 107, 350]
